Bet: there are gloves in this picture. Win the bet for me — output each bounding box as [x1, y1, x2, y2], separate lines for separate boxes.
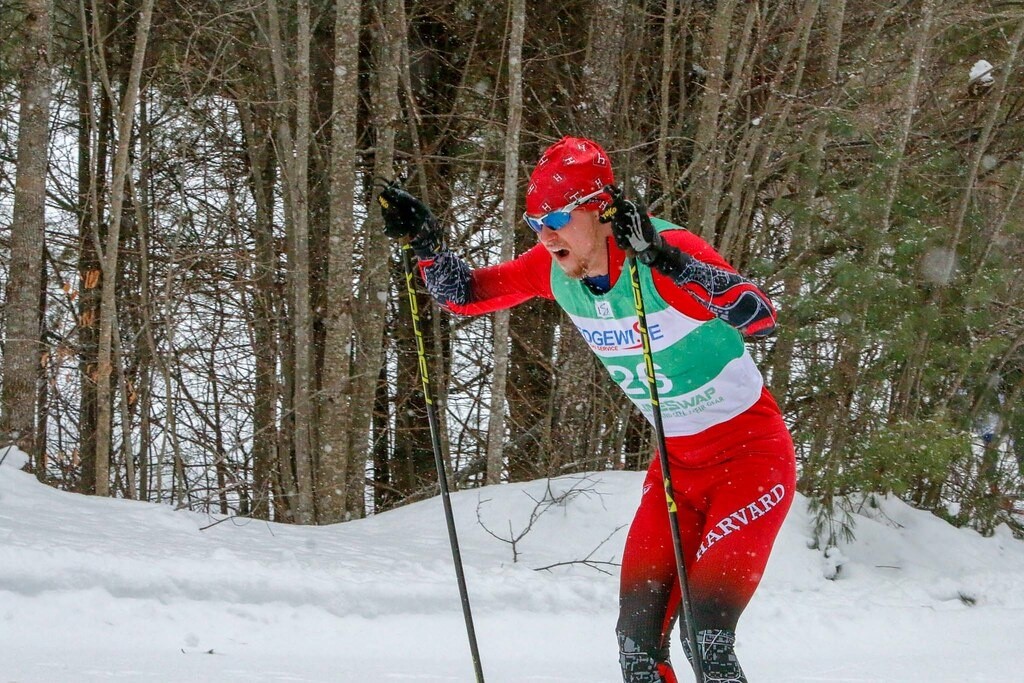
[598, 198, 662, 264]
[377, 189, 443, 257]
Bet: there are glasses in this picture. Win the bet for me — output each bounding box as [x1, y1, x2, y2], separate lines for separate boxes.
[523, 188, 604, 233]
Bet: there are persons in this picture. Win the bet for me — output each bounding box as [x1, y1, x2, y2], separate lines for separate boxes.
[375, 135, 797, 683]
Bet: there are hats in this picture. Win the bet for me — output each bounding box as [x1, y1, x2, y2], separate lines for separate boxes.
[525, 136, 615, 215]
